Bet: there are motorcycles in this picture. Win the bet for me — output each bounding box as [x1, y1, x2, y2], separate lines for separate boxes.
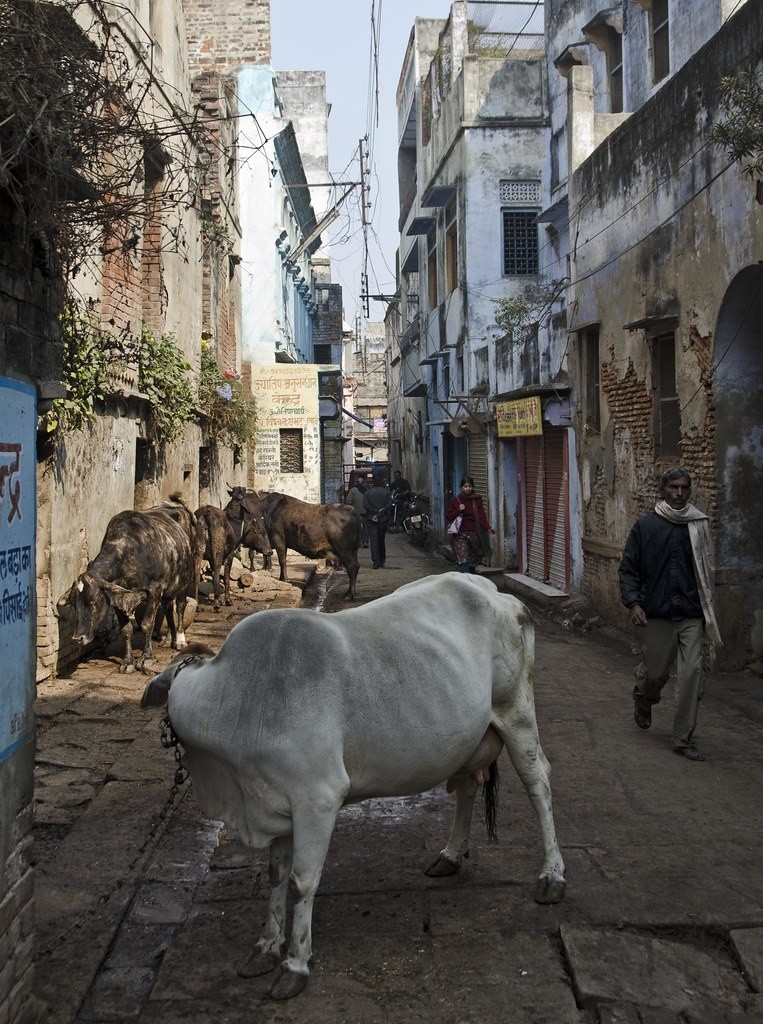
[402, 491, 431, 548]
[384, 488, 407, 533]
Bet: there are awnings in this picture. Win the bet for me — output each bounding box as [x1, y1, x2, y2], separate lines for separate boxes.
[420, 186, 456, 208]
[405, 216, 436, 236]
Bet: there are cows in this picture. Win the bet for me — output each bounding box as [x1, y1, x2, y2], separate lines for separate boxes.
[56, 482, 370, 674]
[138, 570, 565, 1002]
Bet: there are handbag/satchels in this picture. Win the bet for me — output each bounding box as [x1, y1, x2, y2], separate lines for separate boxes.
[447, 495, 464, 535]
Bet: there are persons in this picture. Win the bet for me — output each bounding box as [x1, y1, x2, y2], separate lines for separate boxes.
[391, 471, 411, 494]
[346, 478, 369, 548]
[363, 476, 392, 569]
[617, 468, 725, 761]
[445, 476, 493, 574]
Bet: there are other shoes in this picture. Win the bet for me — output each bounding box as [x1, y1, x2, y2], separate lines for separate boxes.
[372, 564, 386, 569]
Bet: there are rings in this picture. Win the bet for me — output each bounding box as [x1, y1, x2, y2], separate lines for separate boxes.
[634, 622, 637, 625]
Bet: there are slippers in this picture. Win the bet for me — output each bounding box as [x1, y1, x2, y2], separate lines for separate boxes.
[675, 745, 705, 761]
[634, 699, 652, 729]
